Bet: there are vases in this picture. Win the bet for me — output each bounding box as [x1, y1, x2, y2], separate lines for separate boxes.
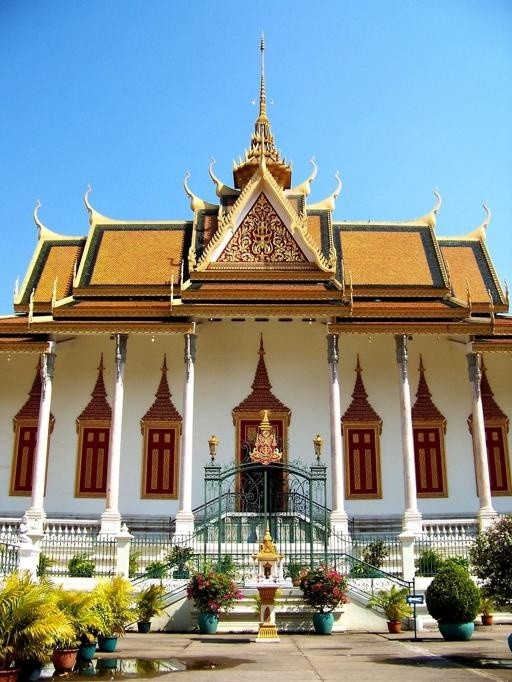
[312, 611, 335, 635]
[197, 614, 218, 633]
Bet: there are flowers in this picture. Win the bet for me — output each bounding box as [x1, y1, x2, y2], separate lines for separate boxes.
[300, 570, 348, 612]
[186, 572, 244, 620]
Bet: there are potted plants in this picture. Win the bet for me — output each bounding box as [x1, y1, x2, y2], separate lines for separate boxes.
[0, 568, 171, 682]
[166, 544, 194, 579]
[283, 561, 302, 586]
[365, 513, 498, 641]
